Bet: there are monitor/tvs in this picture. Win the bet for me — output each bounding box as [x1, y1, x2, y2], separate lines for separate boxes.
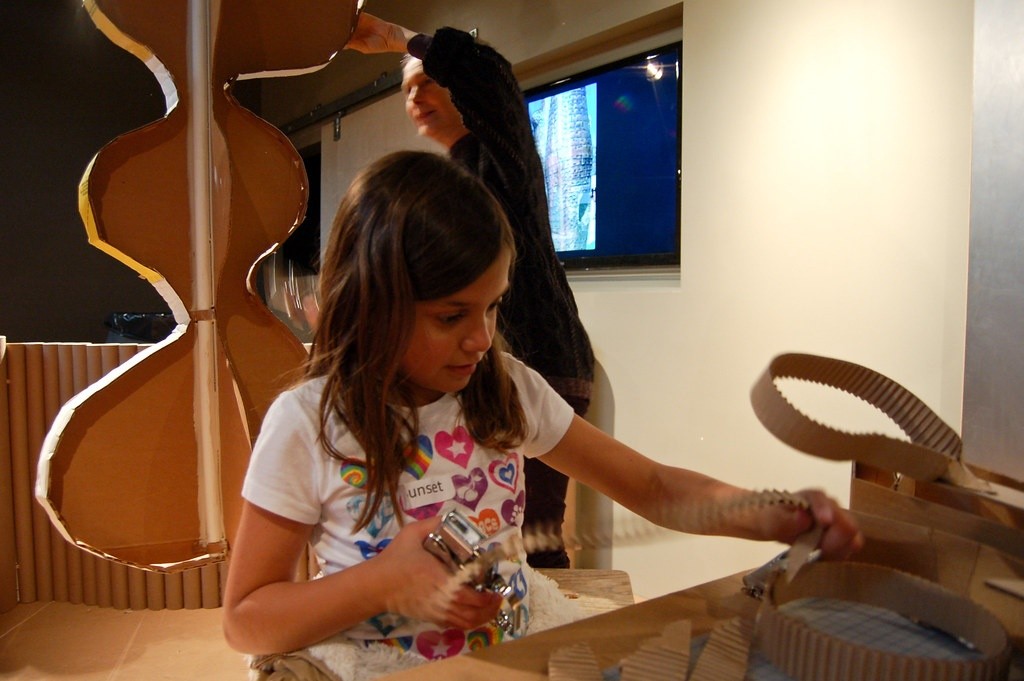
[517, 38, 685, 272]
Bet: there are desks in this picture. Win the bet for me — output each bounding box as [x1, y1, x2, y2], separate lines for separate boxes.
[360, 567, 1024, 681]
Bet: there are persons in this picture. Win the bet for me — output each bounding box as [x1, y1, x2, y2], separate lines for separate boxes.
[344, 11, 596, 570]
[221, 150, 867, 681]
